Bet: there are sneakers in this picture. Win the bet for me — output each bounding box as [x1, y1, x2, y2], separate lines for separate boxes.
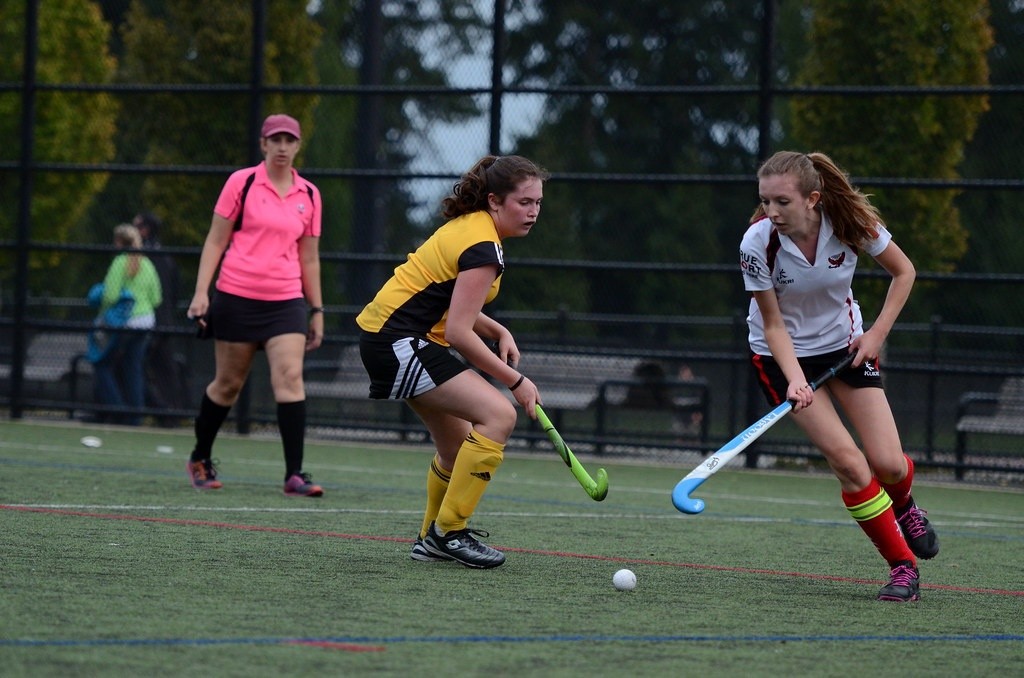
[186, 450, 223, 490]
[878, 560, 919, 602]
[422, 520, 506, 568]
[410, 532, 457, 563]
[894, 495, 939, 560]
[284, 472, 323, 497]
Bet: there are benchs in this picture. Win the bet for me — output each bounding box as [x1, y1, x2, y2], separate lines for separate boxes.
[309, 342, 713, 457]
[952, 377, 1024, 478]
[0, 332, 106, 419]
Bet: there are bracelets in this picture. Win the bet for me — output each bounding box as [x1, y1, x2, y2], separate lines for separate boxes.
[310, 306, 325, 314]
[507, 374, 525, 392]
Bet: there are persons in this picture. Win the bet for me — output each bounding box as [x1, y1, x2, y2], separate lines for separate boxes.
[738, 150, 939, 602]
[133, 210, 176, 425]
[92, 222, 163, 426]
[184, 114, 328, 496]
[672, 366, 713, 436]
[354, 154, 543, 567]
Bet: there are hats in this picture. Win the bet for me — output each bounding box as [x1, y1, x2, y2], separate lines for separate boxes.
[261, 113, 300, 140]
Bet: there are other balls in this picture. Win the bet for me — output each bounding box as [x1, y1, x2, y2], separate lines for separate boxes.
[611, 567, 637, 591]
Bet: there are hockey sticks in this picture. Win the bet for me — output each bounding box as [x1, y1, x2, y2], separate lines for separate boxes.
[492, 338, 609, 501]
[669, 346, 861, 516]
